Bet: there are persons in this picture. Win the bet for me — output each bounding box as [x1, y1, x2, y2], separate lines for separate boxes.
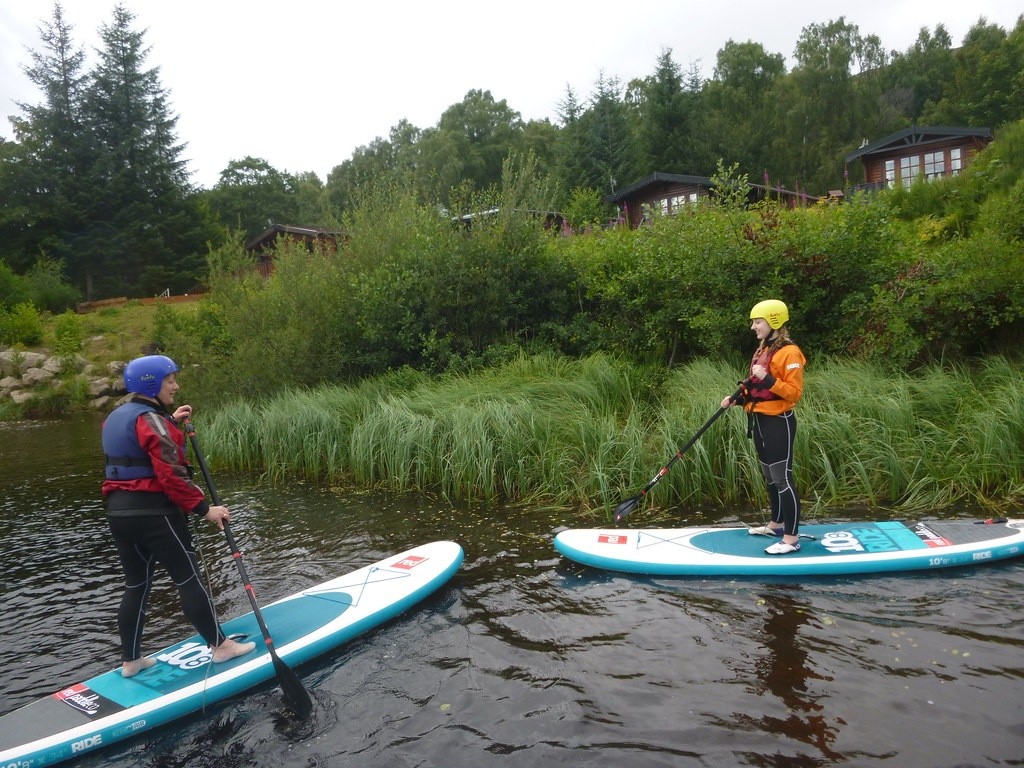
[100, 355, 256, 677]
[720, 300, 806, 555]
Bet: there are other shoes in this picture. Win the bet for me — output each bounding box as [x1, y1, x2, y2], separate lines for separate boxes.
[748, 526, 783, 535]
[764, 541, 800, 554]
[122, 657, 157, 678]
[212, 641, 256, 663]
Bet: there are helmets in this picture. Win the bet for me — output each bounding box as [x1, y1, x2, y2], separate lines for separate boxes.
[750, 299, 789, 329]
[124, 355, 178, 397]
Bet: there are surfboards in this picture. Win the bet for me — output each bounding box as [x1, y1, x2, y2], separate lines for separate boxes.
[553, 518, 1024, 576]
[0, 541, 462, 768]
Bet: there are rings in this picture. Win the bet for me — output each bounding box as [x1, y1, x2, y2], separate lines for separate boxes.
[223, 513, 226, 518]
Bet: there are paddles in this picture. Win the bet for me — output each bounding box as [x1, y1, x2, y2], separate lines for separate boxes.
[183, 415, 311, 711]
[613, 376, 757, 525]
